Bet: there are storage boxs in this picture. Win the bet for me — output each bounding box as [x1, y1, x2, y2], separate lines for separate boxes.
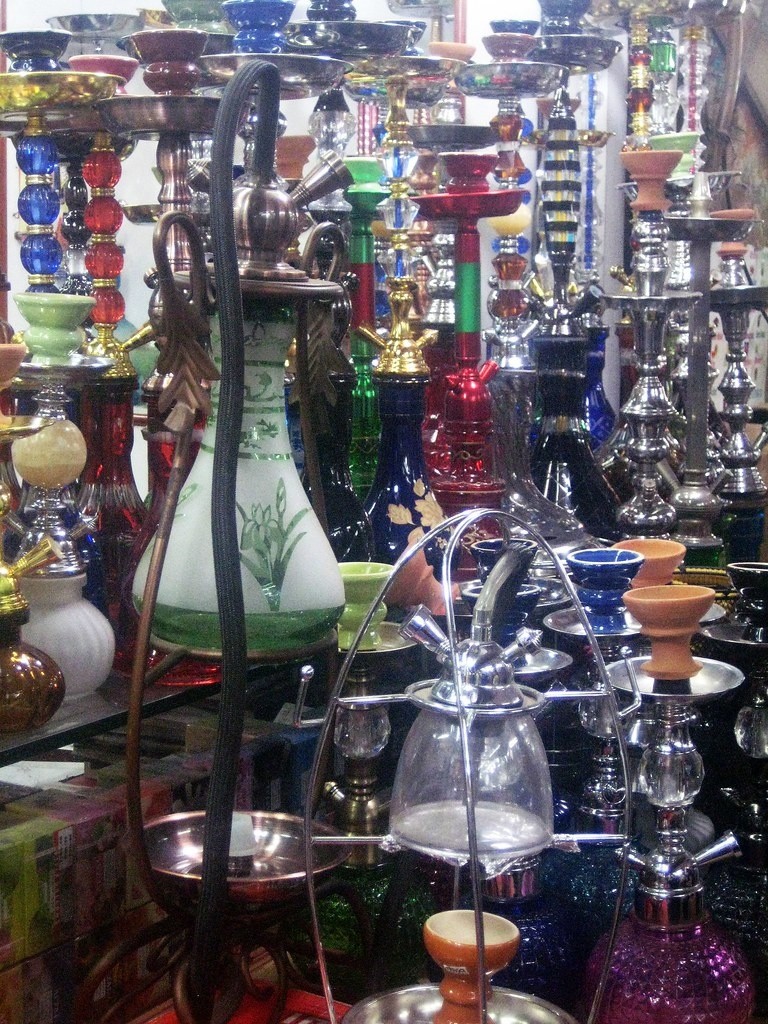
[0, 705, 344, 1024]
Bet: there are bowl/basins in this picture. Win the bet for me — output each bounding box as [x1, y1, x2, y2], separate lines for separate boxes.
[125, 809, 352, 919]
